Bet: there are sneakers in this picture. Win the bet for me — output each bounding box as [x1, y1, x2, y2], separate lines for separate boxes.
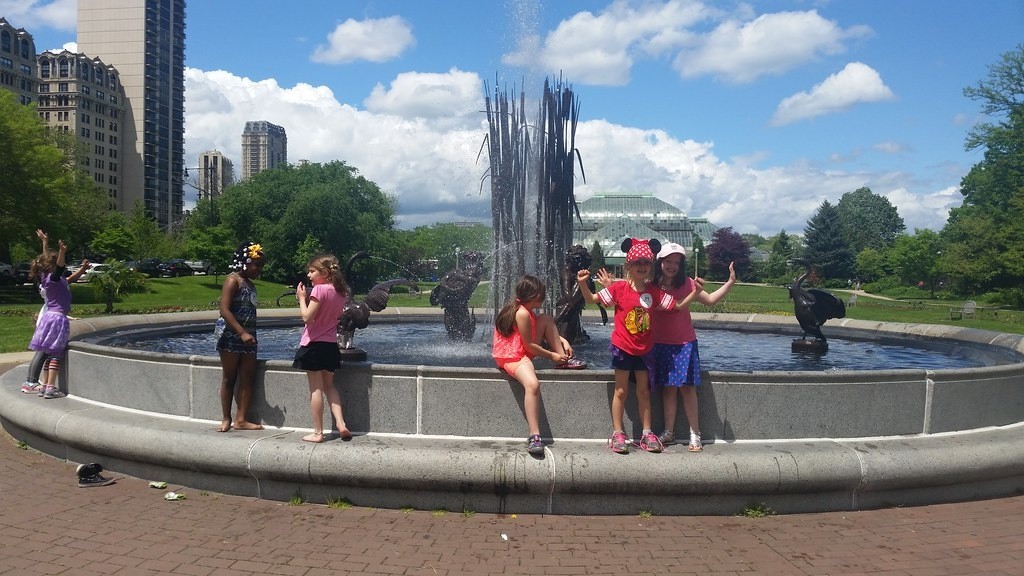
[78, 474, 115, 487]
[554, 357, 587, 369]
[44, 388, 66, 399]
[528, 435, 544, 452]
[610, 433, 638, 453]
[640, 432, 664, 452]
[21, 383, 42, 393]
[76, 462, 103, 476]
[38, 389, 46, 396]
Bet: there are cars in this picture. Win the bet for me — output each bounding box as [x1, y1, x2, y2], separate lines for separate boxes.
[0, 257, 230, 283]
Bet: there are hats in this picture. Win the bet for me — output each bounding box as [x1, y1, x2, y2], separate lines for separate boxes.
[657, 243, 687, 259]
[621, 238, 661, 267]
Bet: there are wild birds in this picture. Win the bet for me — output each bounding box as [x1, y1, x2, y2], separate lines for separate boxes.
[430, 251, 488, 304]
[786, 258, 845, 342]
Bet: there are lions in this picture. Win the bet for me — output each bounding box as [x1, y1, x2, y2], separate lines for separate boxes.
[564, 244, 593, 270]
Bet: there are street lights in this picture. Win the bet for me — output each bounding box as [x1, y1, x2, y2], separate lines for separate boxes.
[455, 247, 461, 269]
[183, 160, 216, 226]
[694, 248, 699, 278]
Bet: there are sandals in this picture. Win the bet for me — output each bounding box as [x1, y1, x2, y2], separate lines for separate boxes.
[658, 430, 676, 445]
[688, 434, 703, 452]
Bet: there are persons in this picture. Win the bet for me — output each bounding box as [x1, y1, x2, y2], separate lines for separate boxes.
[855, 276, 859, 291]
[596, 242, 735, 452]
[848, 277, 851, 289]
[577, 238, 705, 453]
[291, 255, 351, 443]
[29, 229, 71, 397]
[21, 259, 92, 394]
[492, 275, 587, 453]
[213, 242, 266, 431]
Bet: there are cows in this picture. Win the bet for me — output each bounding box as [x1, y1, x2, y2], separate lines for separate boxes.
[335, 285, 372, 349]
